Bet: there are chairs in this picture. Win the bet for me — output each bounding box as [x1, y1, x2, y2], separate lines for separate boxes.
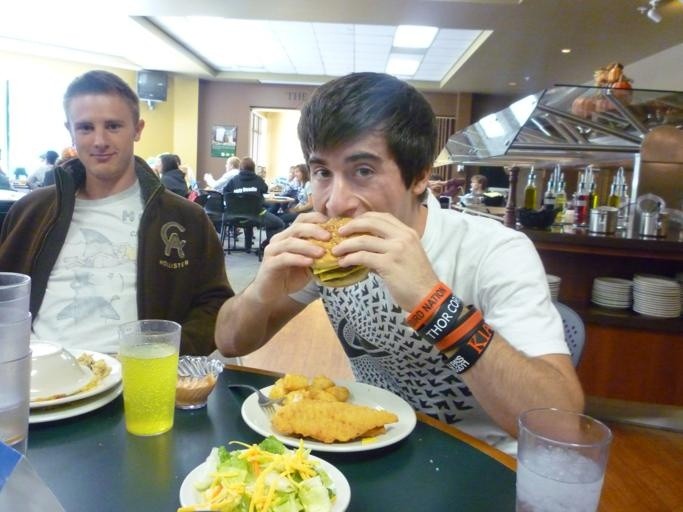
[195, 189, 231, 256]
[550, 299, 588, 371]
[221, 191, 262, 262]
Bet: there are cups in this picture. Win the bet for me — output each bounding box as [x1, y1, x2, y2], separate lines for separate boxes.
[118, 319, 225, 437]
[20, 174, 26, 185]
[515, 407, 613, 512]
[215, 128, 234, 143]
[0, 271, 32, 456]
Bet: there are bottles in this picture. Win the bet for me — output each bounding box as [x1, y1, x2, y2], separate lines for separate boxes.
[524, 164, 671, 237]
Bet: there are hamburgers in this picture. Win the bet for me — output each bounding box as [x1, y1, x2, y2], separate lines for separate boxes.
[306, 217, 368, 288]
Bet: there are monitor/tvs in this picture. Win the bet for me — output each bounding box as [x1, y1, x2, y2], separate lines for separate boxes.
[136, 69, 167, 102]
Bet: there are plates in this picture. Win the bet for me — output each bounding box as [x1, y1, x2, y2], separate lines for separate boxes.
[14, 186, 29, 188]
[179, 450, 351, 512]
[546, 273, 682, 319]
[240, 380, 417, 452]
[29, 342, 122, 423]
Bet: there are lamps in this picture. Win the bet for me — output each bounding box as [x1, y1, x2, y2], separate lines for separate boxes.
[643, 0, 663, 23]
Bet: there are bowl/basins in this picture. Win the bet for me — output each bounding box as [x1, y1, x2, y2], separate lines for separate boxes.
[458, 196, 485, 205]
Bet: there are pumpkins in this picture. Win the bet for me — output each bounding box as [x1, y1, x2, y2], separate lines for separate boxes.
[572, 64, 633, 117]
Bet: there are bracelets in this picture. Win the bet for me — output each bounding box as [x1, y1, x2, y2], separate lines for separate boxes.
[404, 282, 494, 374]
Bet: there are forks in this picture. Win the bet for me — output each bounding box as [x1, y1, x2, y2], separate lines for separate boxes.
[229, 383, 276, 414]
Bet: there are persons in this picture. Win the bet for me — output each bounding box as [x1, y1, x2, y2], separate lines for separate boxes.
[0, 69, 235, 359]
[215, 72, 585, 460]
